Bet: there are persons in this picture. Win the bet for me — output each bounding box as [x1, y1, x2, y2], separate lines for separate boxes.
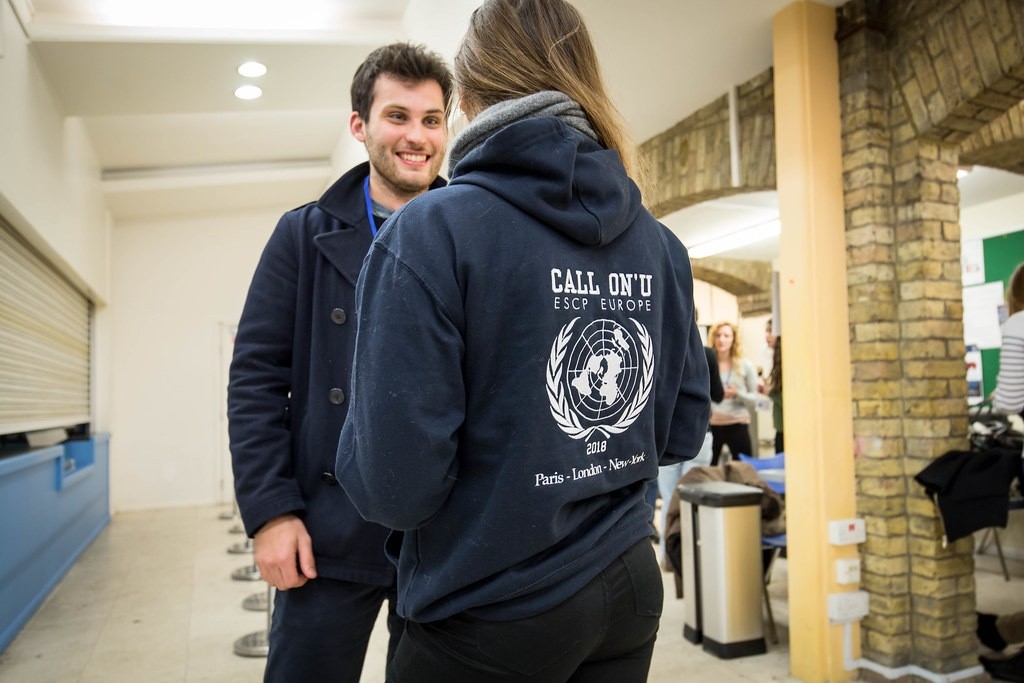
[710, 319, 759, 468]
[756, 317, 785, 456]
[987, 260, 1024, 422]
[225, 42, 454, 683]
[659, 308, 725, 574]
[333, 1, 712, 682]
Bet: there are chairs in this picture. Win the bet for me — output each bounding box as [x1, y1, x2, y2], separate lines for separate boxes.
[737, 452, 788, 646]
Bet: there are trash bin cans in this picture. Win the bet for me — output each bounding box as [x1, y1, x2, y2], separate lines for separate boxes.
[677, 480, 766, 660]
